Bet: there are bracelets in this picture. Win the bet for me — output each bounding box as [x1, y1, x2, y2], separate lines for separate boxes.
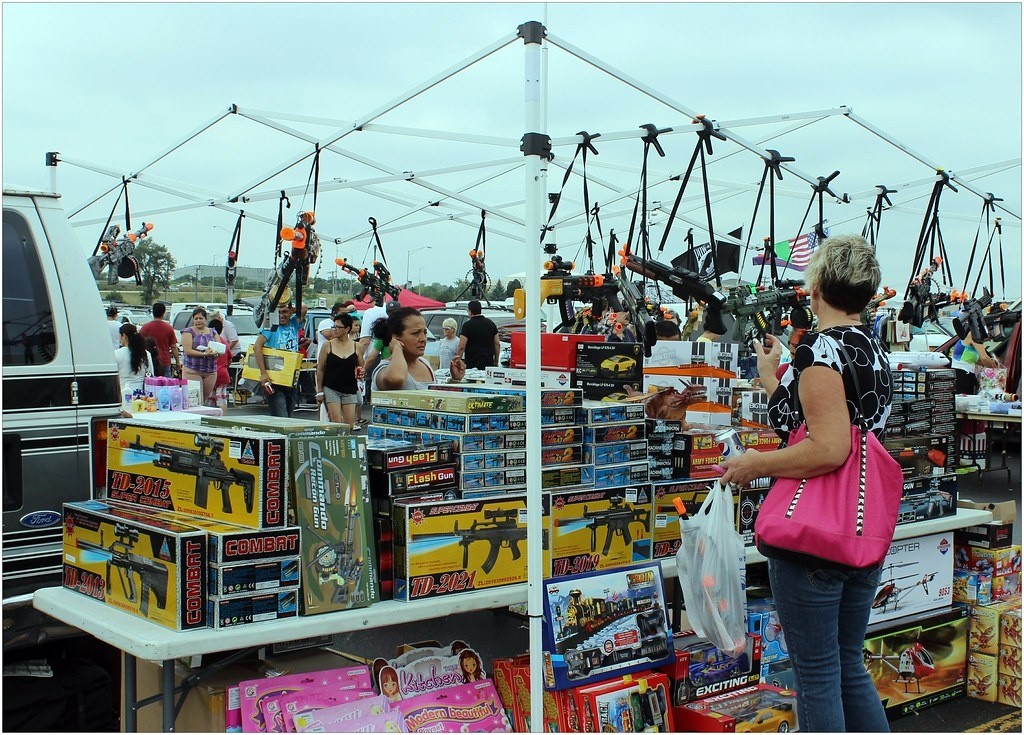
[317, 391, 323, 393]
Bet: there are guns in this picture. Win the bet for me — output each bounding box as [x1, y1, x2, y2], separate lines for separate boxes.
[415, 486, 707, 572]
[114, 434, 254, 518]
[665, 494, 747, 654]
[308, 505, 366, 607]
[88, 221, 1023, 358]
[68, 525, 169, 620]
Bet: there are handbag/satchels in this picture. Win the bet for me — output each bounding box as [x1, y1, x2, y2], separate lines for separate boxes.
[143, 357, 153, 393]
[676, 479, 747, 658]
[754, 336, 904, 574]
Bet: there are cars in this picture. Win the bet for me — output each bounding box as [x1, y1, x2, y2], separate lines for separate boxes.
[910, 298, 1022, 352]
[102, 284, 703, 370]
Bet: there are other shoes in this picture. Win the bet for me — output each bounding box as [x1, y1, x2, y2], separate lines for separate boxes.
[352, 425, 362, 430]
[356, 418, 369, 425]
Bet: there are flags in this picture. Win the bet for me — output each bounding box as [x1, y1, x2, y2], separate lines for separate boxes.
[670, 227, 744, 287]
[752, 227, 831, 271]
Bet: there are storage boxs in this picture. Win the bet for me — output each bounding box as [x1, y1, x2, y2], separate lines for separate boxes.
[65, 336, 1022, 733]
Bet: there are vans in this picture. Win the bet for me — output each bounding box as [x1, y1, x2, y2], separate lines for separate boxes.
[2, 186, 123, 733]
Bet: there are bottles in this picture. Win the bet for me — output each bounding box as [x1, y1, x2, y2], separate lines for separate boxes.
[131, 376, 190, 414]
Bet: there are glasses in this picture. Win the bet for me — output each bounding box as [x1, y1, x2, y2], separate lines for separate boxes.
[443, 328, 452, 330]
[331, 326, 344, 330]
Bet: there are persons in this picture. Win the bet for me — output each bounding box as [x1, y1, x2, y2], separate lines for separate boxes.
[952, 330, 995, 394]
[314, 301, 389, 431]
[439, 317, 460, 368]
[457, 300, 500, 370]
[652, 321, 681, 341]
[106, 301, 242, 412]
[719, 233, 892, 733]
[696, 320, 728, 342]
[255, 295, 312, 417]
[370, 307, 466, 392]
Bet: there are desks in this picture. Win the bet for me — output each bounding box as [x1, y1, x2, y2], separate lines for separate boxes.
[965, 413, 1022, 487]
[227, 365, 319, 412]
[31, 508, 992, 733]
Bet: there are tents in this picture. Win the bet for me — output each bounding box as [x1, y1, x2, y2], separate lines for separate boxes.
[352, 284, 446, 311]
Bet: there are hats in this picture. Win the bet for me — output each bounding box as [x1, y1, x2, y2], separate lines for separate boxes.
[107, 307, 119, 315]
[386, 301, 400, 311]
